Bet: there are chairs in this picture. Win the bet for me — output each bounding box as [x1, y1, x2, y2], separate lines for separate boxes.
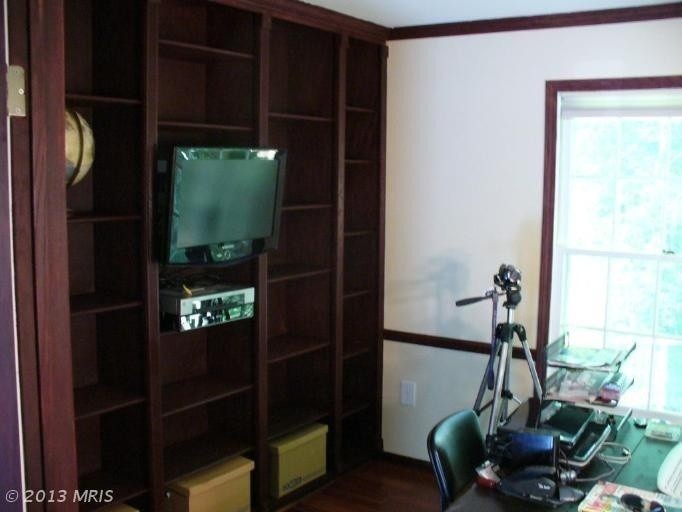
[427, 409, 490, 512]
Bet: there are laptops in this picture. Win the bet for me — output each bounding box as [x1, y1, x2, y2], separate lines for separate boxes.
[499, 399, 613, 462]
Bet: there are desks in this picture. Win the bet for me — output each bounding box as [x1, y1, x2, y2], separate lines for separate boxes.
[446, 396, 682, 512]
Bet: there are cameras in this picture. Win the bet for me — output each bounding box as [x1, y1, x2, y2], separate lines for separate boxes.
[494, 263, 524, 308]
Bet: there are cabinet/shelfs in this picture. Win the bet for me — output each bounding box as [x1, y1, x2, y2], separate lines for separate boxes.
[0, 0, 154, 512]
[154, 1, 265, 512]
[339, 16, 385, 468]
[264, 0, 340, 509]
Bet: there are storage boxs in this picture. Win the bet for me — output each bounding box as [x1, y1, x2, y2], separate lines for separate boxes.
[163, 456, 254, 512]
[266, 422, 329, 499]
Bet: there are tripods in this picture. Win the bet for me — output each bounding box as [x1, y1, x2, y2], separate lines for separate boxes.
[472, 321, 543, 455]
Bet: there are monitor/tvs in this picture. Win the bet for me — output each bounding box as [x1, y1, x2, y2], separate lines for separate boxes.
[163, 146, 289, 265]
